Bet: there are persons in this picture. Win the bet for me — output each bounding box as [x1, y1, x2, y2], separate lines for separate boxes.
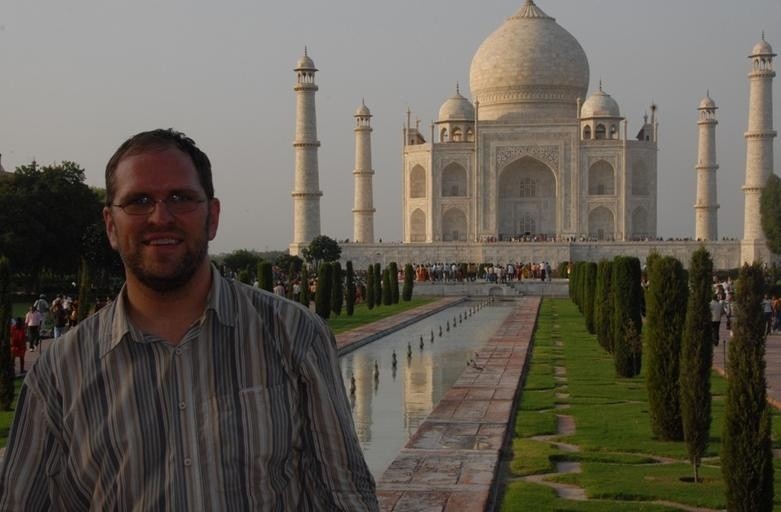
[11, 317, 28, 372]
[412, 261, 553, 283]
[355, 284, 363, 304]
[96, 297, 105, 313]
[640, 275, 650, 317]
[293, 276, 319, 300]
[254, 278, 259, 287]
[479, 234, 737, 242]
[25, 291, 79, 352]
[0, 127, 380, 512]
[708, 276, 781, 346]
[274, 279, 288, 296]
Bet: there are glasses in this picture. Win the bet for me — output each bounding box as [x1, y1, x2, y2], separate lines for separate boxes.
[108, 192, 207, 214]
